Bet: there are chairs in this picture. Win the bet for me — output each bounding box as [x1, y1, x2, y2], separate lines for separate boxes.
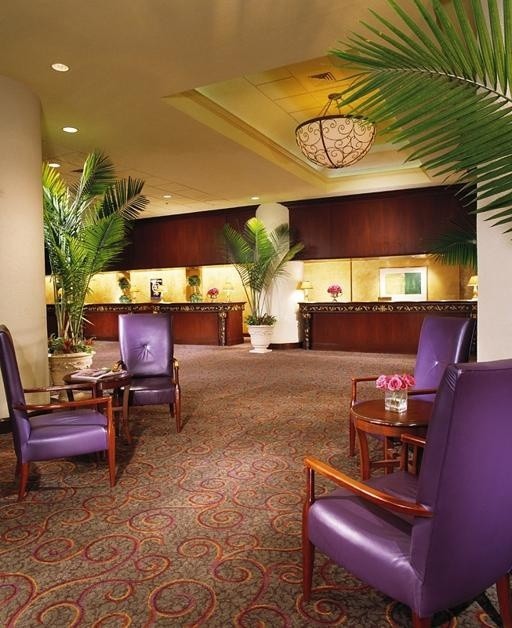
[116, 312, 183, 437]
[0, 323, 118, 502]
[299, 356, 512, 628]
[346, 312, 476, 458]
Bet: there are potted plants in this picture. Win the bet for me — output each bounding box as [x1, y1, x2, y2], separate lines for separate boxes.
[40, 144, 150, 389]
[210, 217, 305, 354]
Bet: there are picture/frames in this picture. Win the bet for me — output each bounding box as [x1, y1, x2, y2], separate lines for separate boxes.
[377, 265, 430, 304]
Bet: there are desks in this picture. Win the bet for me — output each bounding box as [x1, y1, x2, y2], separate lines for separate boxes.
[62, 367, 133, 461]
[348, 398, 436, 482]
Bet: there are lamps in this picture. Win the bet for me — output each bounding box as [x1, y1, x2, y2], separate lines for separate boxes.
[294, 92, 377, 170]
[223, 282, 235, 302]
[466, 275, 477, 300]
[156, 285, 168, 304]
[299, 280, 314, 302]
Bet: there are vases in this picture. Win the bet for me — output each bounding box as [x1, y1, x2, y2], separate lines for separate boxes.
[380, 390, 408, 413]
[329, 292, 339, 303]
[210, 295, 218, 303]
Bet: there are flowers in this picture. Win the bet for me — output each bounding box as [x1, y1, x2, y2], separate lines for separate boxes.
[206, 287, 220, 297]
[326, 283, 341, 293]
[373, 371, 417, 393]
